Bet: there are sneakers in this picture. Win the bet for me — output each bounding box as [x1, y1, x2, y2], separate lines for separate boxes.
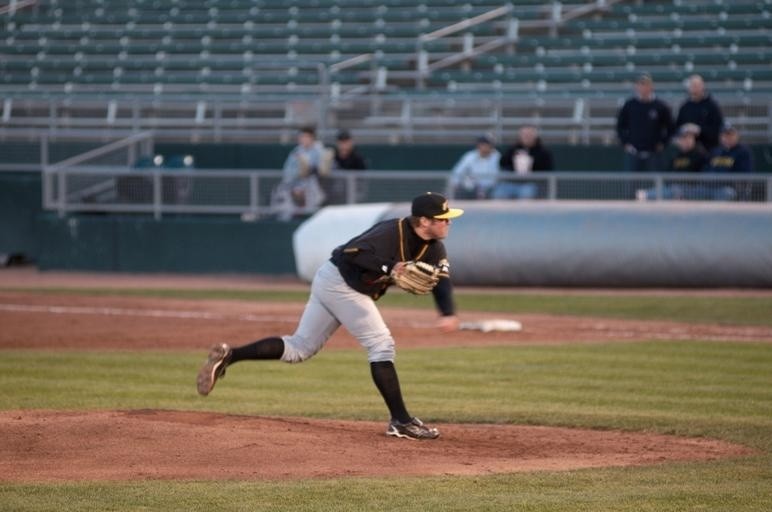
[387, 417, 439, 440]
[197, 342, 232, 396]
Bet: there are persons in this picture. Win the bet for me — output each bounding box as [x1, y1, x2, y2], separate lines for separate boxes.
[678, 74, 725, 152]
[199, 191, 457, 443]
[493, 123, 556, 199]
[333, 128, 365, 169]
[269, 126, 337, 209]
[681, 123, 755, 201]
[448, 133, 501, 201]
[635, 115, 705, 199]
[615, 70, 674, 199]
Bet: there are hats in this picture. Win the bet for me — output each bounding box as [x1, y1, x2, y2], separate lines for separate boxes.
[412, 192, 464, 219]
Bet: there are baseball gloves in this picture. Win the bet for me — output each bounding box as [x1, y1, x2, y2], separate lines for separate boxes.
[392, 262, 438, 295]
